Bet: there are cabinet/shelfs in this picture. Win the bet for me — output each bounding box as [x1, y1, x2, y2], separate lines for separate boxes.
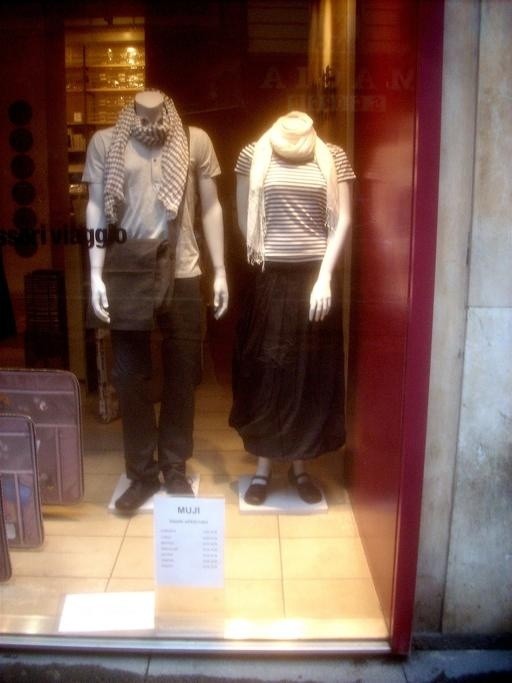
[66, 38, 148, 202]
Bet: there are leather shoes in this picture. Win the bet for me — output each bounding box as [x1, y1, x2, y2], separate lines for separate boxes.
[245, 472, 271, 505]
[289, 465, 320, 503]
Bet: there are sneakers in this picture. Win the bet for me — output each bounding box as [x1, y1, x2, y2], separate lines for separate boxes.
[161, 466, 194, 498]
[115, 475, 160, 513]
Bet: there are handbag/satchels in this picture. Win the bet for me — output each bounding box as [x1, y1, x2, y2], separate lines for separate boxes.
[85, 235, 176, 332]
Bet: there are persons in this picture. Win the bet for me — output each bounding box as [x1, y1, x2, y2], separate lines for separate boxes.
[234, 110, 357, 506]
[80, 88, 231, 508]
